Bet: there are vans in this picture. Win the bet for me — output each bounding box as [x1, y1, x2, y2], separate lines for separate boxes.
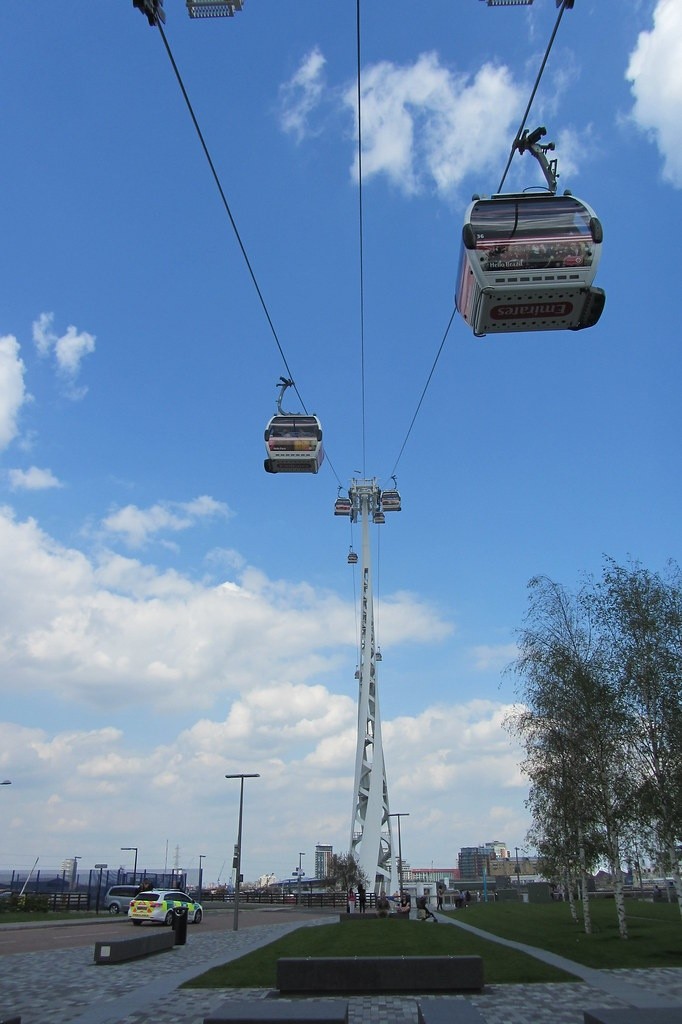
[105, 885, 144, 916]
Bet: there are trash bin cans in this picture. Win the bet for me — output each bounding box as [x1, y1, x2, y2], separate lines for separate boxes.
[171, 907, 189, 945]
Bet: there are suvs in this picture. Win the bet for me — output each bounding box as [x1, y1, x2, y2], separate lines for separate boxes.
[0, 779, 12, 785]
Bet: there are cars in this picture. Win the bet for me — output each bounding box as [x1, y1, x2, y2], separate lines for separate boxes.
[128, 890, 203, 925]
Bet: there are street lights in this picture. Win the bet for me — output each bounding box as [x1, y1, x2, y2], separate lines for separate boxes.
[65, 856, 80, 911]
[514, 848, 520, 899]
[388, 813, 410, 906]
[226, 773, 260, 931]
[121, 847, 138, 884]
[297, 853, 305, 904]
[198, 855, 206, 904]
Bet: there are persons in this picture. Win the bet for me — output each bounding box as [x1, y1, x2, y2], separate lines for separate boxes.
[138, 878, 153, 892]
[358, 884, 366, 914]
[347, 888, 356, 914]
[393, 891, 410, 907]
[454, 889, 472, 909]
[548, 882, 565, 899]
[437, 886, 444, 911]
[376, 891, 390, 917]
